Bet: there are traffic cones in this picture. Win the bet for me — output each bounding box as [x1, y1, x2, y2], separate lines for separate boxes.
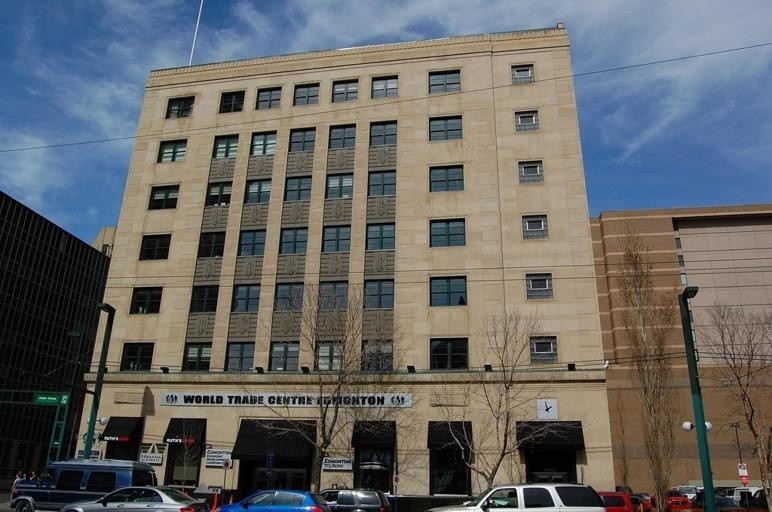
[228, 494, 234, 504]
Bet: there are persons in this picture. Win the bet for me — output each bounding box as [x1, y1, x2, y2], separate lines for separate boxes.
[15, 470, 51, 481]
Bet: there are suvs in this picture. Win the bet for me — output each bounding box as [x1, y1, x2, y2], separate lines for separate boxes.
[426, 480, 606, 512]
[318, 487, 392, 511]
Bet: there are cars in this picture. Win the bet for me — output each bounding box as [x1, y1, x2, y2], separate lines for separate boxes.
[57, 484, 211, 512]
[211, 490, 331, 512]
[595, 480, 771, 512]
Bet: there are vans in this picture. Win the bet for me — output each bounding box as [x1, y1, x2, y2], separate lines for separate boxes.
[249, 466, 309, 493]
[8, 458, 157, 511]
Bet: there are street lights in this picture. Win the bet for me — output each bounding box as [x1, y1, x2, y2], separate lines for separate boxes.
[83, 303, 117, 460]
[55, 330, 86, 461]
[677, 287, 716, 512]
[725, 422, 746, 486]
